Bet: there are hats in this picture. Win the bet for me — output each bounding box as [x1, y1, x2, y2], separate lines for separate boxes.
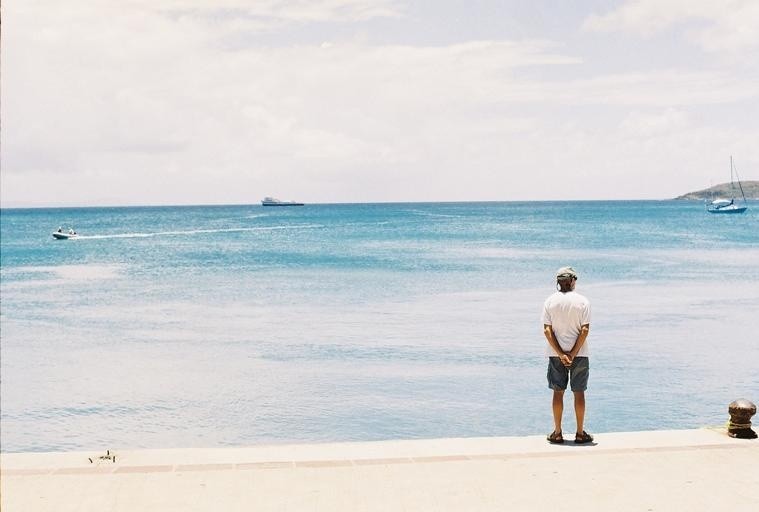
[556, 267, 576, 281]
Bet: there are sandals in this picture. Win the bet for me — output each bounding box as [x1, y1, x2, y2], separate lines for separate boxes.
[547, 429, 562, 442]
[574, 430, 593, 443]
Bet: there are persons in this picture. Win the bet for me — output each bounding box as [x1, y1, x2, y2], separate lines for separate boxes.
[541, 266, 593, 443]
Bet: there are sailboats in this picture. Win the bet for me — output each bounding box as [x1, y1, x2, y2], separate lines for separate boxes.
[708, 156, 748, 213]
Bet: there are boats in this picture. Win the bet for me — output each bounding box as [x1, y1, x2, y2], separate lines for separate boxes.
[261, 196, 304, 206]
[53, 232, 79, 239]
